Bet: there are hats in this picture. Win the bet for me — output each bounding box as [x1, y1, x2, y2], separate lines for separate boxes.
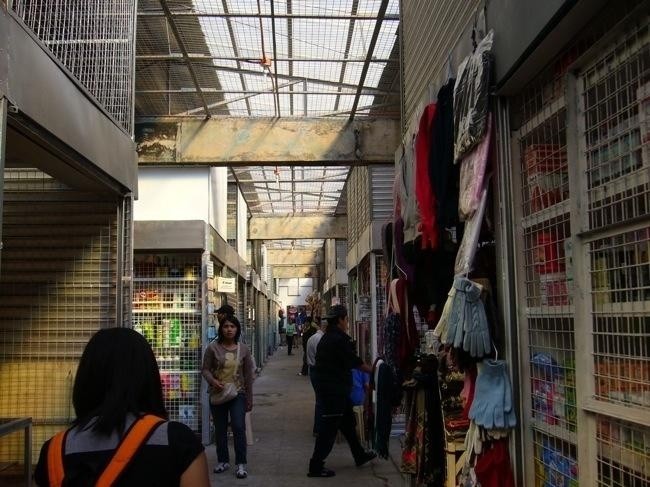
[321, 304, 348, 321]
[214, 305, 235, 314]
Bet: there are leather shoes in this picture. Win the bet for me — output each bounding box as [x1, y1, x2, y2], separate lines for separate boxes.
[307, 458, 336, 478]
[353, 448, 377, 467]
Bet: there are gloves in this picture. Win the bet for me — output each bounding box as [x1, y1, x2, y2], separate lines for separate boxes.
[432, 274, 518, 484]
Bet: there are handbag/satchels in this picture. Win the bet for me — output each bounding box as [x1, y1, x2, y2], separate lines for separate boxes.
[210, 382, 239, 406]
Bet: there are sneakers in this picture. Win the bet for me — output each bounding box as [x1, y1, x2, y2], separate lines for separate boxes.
[213, 461, 232, 473]
[235, 462, 249, 478]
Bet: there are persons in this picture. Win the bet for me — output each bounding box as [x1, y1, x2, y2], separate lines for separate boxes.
[351, 368, 369, 405]
[307, 305, 374, 478]
[295, 316, 317, 376]
[34, 328, 210, 487]
[286, 319, 299, 356]
[306, 321, 328, 437]
[201, 305, 254, 478]
